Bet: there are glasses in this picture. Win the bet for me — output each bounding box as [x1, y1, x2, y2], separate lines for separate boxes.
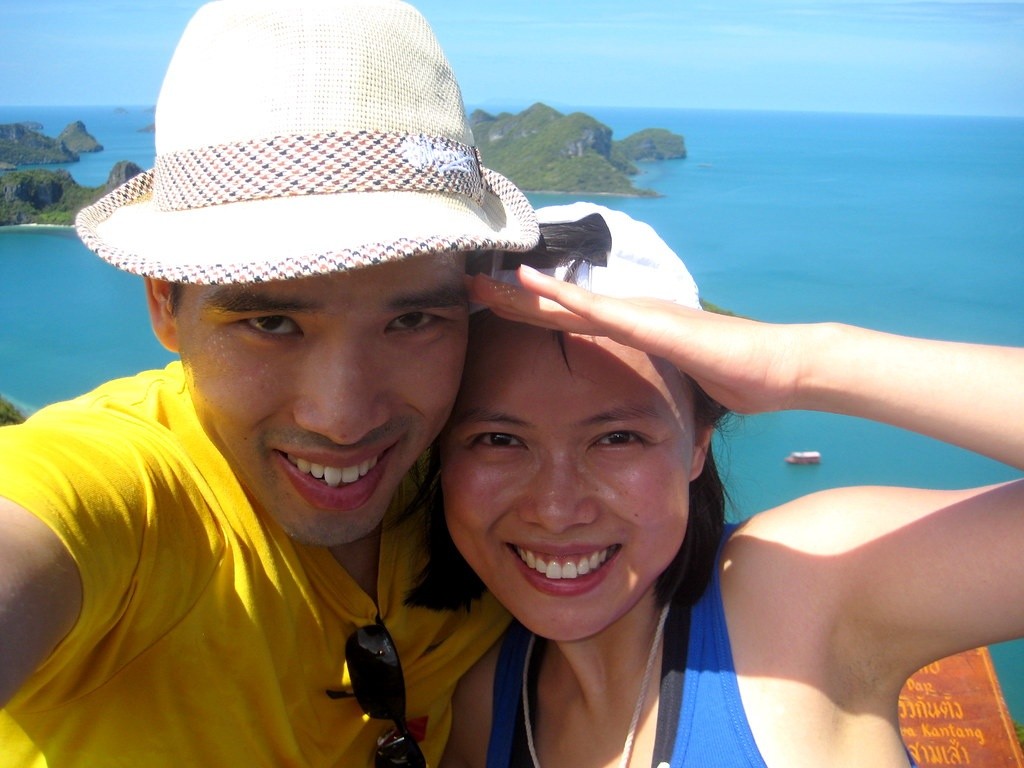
[326, 613, 426, 768]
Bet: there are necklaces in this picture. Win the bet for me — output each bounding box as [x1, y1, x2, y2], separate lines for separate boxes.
[521, 602, 667, 768]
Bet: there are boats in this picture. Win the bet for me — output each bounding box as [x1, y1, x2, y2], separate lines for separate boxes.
[786, 451, 821, 463]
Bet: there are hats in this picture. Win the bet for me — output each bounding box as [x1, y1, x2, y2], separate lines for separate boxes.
[74, 0, 540, 285]
[466, 201, 704, 314]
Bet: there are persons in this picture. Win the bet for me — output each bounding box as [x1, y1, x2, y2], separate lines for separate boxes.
[0, 0, 1024, 768]
